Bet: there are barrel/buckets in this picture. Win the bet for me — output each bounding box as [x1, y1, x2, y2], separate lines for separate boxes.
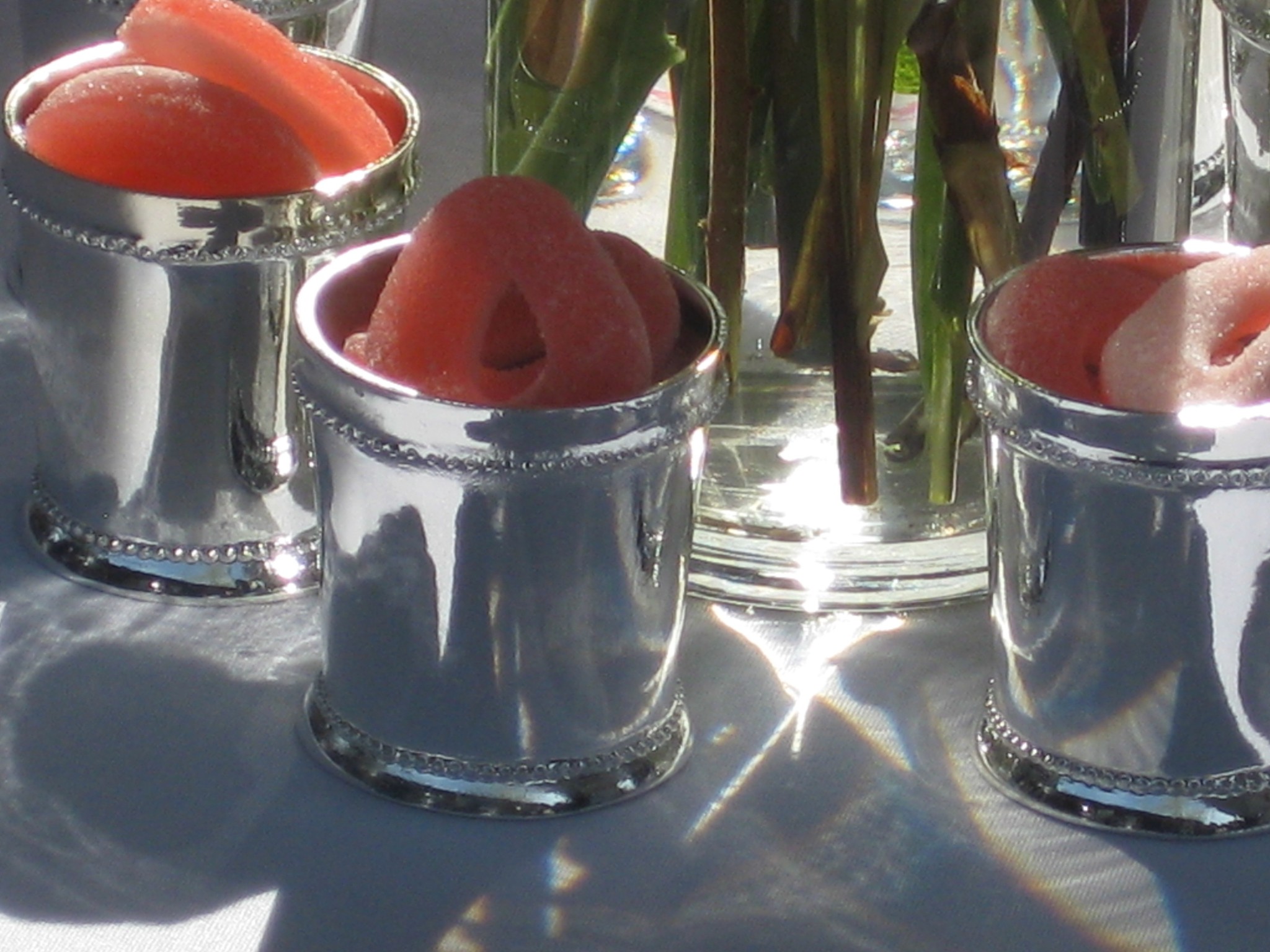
[967, 243, 1270, 836]
[291, 232, 729, 819]
[7, 41, 420, 607]
